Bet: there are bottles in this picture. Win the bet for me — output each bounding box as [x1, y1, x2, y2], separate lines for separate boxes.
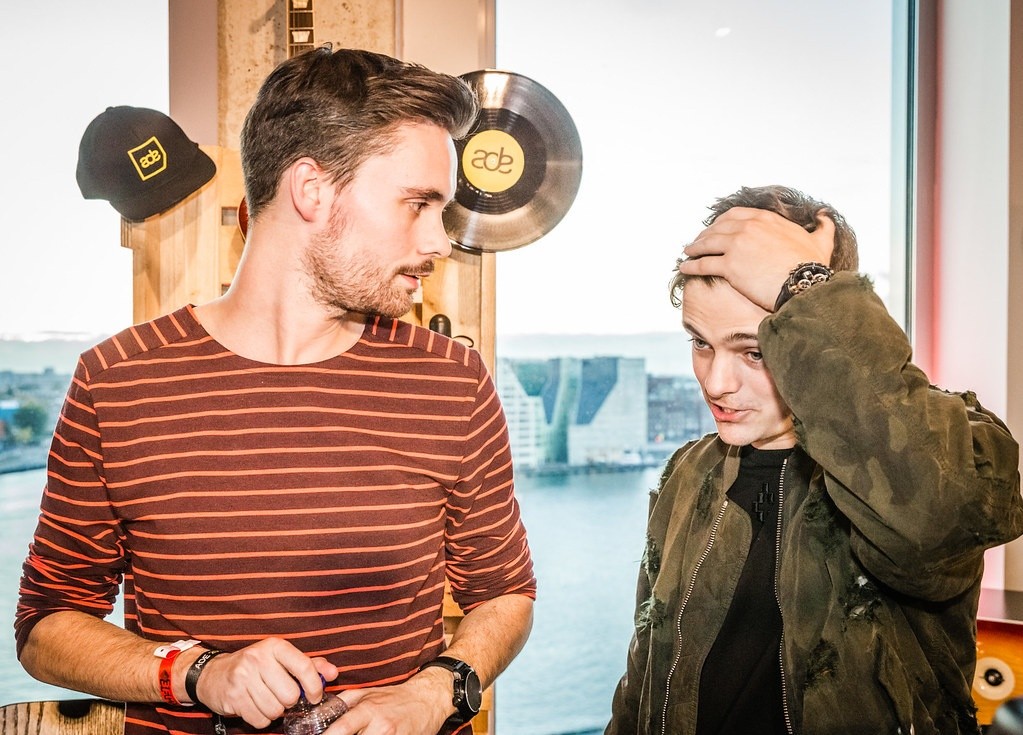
[282, 672, 358, 735]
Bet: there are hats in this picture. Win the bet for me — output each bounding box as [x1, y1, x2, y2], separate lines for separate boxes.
[76, 105, 216, 222]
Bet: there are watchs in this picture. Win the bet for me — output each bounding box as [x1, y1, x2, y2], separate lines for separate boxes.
[773, 262, 835, 313]
[419, 656, 482, 730]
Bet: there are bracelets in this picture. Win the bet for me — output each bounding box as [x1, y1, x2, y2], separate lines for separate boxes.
[158, 639, 201, 707]
[185, 648, 225, 709]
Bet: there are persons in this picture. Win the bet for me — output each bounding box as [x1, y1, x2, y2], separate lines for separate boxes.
[602, 186, 1023, 735]
[13, 46, 537, 735]
